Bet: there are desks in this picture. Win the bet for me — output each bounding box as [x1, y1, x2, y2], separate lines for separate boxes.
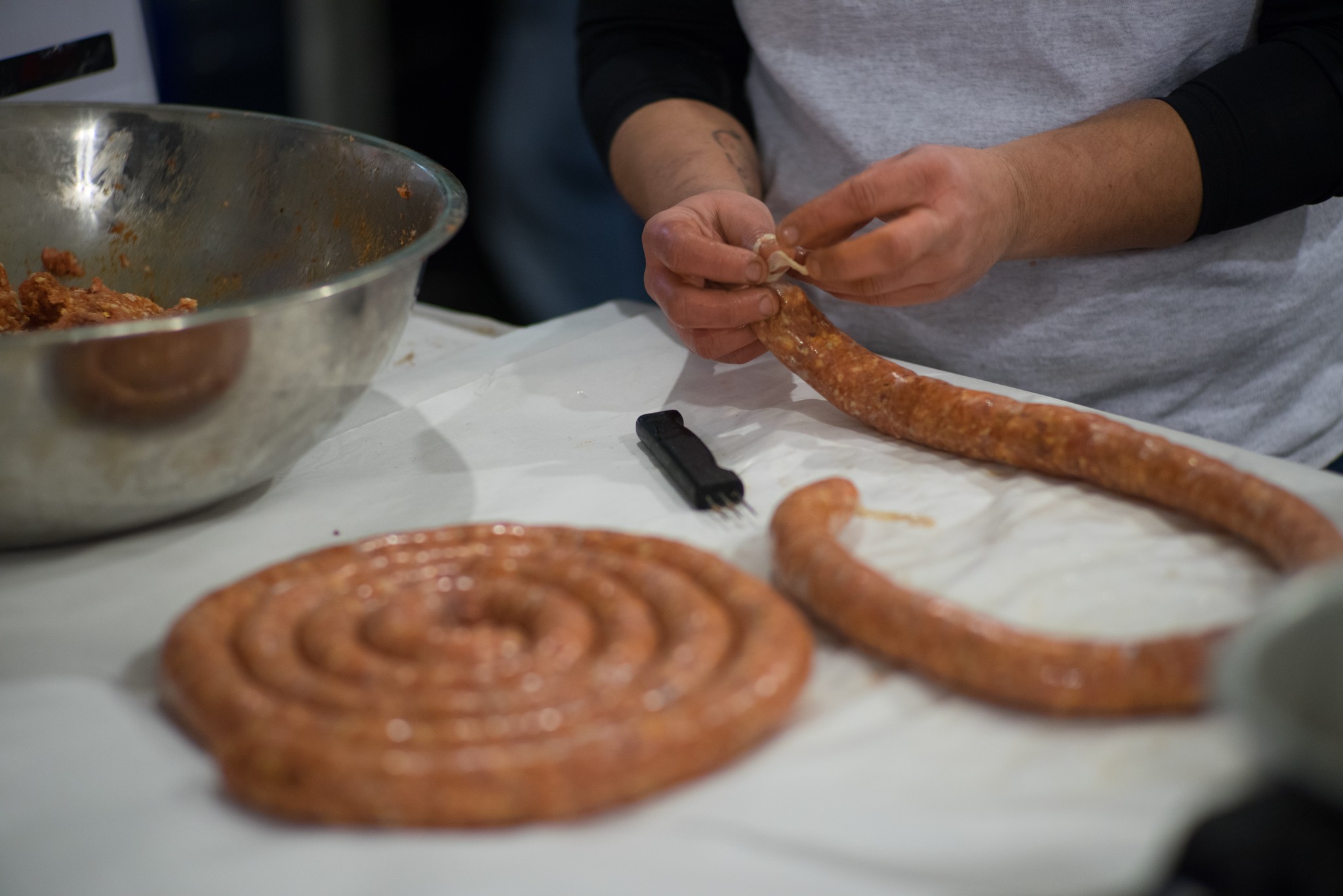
[0, 302, 1343, 896]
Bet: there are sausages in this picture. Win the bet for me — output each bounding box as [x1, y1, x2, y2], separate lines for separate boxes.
[726, 279, 1343, 713]
[152, 525, 813, 832]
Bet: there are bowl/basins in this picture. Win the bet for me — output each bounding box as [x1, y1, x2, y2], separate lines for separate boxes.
[1, 100, 466, 549]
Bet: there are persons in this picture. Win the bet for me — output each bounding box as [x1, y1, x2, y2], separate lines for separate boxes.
[577, 0, 1343, 474]
